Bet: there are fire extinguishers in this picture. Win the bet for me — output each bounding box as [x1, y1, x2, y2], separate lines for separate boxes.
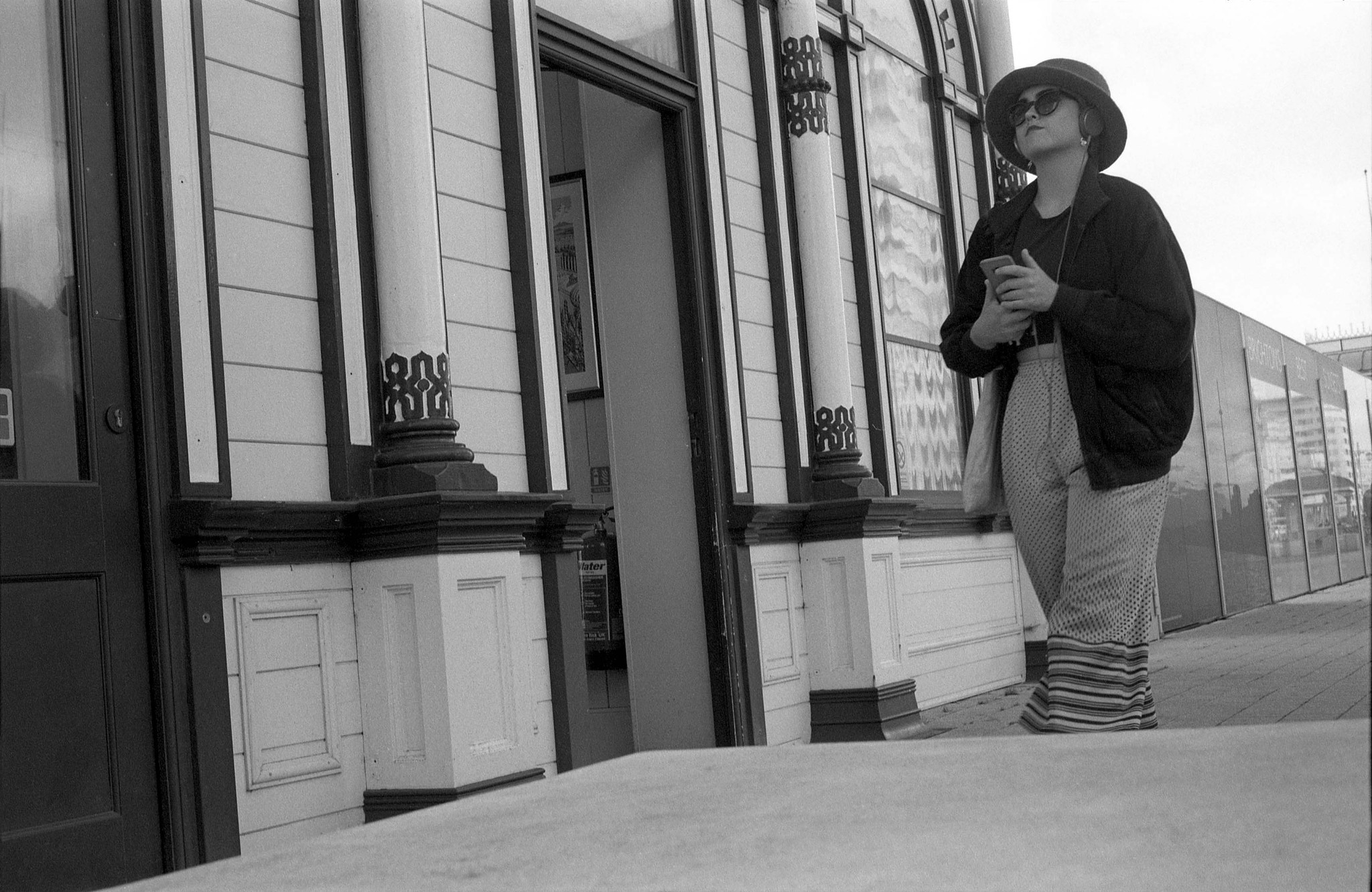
[579, 505, 629, 670]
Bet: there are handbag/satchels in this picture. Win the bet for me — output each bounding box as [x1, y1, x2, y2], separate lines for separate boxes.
[959, 364, 1010, 517]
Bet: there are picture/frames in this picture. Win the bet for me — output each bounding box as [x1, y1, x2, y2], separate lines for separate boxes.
[549, 170, 604, 402]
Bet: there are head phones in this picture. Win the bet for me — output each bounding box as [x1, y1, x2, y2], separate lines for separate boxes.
[1010, 100, 1105, 156]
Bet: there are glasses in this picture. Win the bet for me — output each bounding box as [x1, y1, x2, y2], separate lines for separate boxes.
[1005, 88, 1084, 130]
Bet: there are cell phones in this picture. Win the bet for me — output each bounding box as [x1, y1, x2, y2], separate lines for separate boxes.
[980, 255, 1017, 304]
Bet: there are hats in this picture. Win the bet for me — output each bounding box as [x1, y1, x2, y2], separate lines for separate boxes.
[985, 58, 1128, 176]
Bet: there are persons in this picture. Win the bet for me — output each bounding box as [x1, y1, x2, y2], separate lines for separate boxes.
[940, 58, 1196, 734]
